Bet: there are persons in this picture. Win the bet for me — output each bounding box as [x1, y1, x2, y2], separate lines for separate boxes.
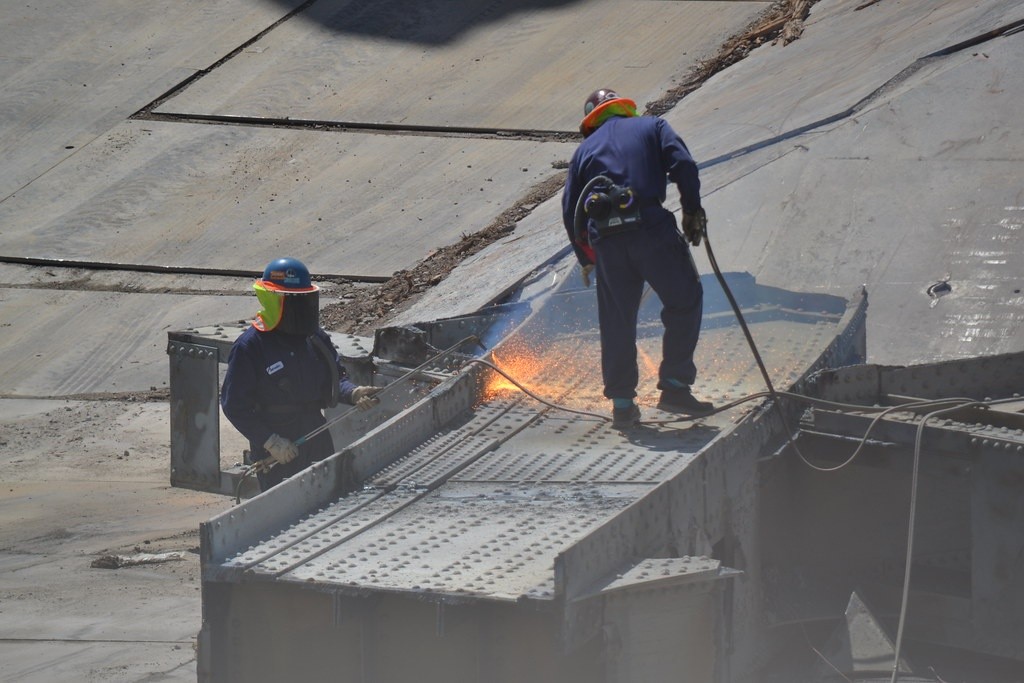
[217, 258, 385, 495]
[561, 85, 717, 428]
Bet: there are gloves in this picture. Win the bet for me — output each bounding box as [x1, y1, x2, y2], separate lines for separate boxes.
[581, 264, 596, 287]
[351, 385, 384, 411]
[262, 434, 298, 464]
[684, 210, 706, 247]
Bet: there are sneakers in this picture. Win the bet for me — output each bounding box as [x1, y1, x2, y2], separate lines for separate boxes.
[612, 404, 641, 426]
[657, 391, 713, 415]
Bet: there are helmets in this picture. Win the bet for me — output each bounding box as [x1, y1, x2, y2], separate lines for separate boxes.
[252, 256, 319, 294]
[578, 89, 637, 134]
